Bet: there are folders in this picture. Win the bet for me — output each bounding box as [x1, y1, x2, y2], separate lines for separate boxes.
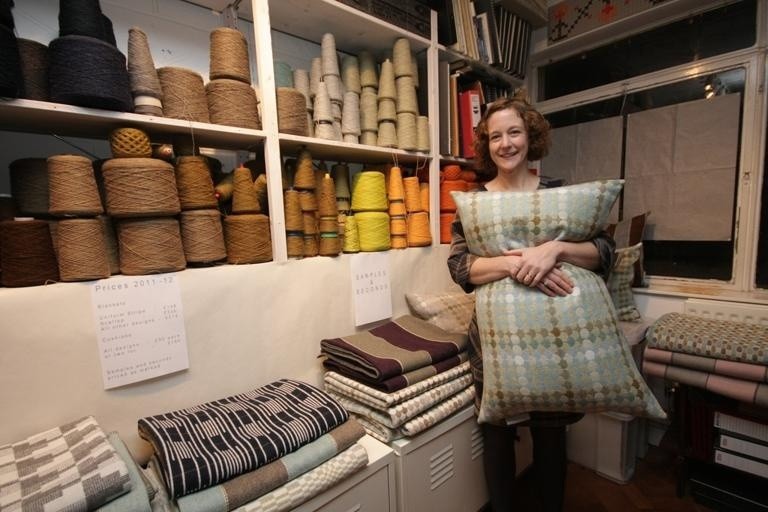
[458, 90, 483, 159]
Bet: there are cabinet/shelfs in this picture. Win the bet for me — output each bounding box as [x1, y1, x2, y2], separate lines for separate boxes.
[558, 314, 655, 486]
[290, 433, 399, 512]
[666, 383, 767, 511]
[390, 403, 492, 512]
[0, 0, 547, 466]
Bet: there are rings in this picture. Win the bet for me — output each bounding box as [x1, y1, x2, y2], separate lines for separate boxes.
[527, 272, 534, 279]
[544, 280, 549, 286]
[513, 263, 520, 270]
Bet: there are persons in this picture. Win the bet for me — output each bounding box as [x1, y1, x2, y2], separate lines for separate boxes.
[445, 95, 619, 512]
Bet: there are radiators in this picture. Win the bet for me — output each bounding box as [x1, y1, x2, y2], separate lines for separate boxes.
[683, 296, 768, 322]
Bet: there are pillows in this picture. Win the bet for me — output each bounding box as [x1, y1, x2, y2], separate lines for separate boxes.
[607, 242, 645, 323]
[449, 178, 669, 424]
[607, 211, 651, 287]
[404, 292, 474, 335]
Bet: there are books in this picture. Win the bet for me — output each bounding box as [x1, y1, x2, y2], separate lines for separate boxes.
[437, 0, 532, 161]
